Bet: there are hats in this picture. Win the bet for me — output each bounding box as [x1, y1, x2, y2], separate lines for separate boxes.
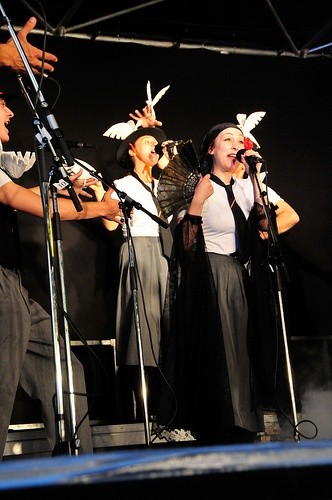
[115, 127, 166, 165]
[202, 122, 245, 152]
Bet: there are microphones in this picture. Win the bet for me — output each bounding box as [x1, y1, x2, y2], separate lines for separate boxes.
[155, 138, 191, 155]
[236, 149, 265, 163]
[45, 141, 94, 152]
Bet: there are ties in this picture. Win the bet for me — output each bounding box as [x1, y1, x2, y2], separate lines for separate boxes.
[130, 172, 176, 261]
[207, 172, 249, 264]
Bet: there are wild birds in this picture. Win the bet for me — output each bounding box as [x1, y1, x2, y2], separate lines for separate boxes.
[236, 112, 267, 149]
[105, 80, 169, 139]
[0, 151, 36, 179]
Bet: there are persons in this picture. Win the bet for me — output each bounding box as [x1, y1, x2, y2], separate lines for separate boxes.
[88, 127, 186, 423]
[126, 106, 299, 239]
[0, 88, 134, 466]
[177, 121, 268, 441]
[0, 17, 58, 77]
